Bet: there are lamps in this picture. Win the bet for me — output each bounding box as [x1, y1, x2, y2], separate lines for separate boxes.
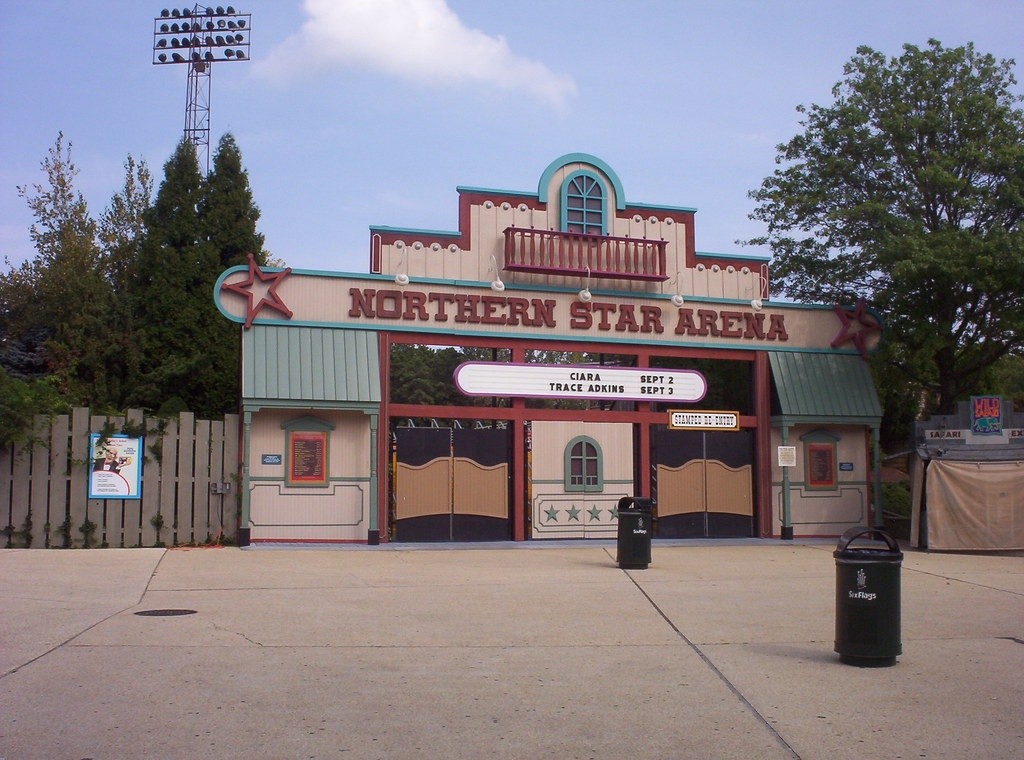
[670, 271, 685, 306]
[395, 246, 410, 285]
[488, 254, 505, 292]
[578, 265, 592, 302]
[744, 276, 767, 311]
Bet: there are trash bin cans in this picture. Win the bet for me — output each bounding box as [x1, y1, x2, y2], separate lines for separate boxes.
[830, 524, 905, 667]
[616, 497, 655, 568]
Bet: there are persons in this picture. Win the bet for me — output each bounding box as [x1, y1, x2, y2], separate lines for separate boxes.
[92, 448, 131, 475]
